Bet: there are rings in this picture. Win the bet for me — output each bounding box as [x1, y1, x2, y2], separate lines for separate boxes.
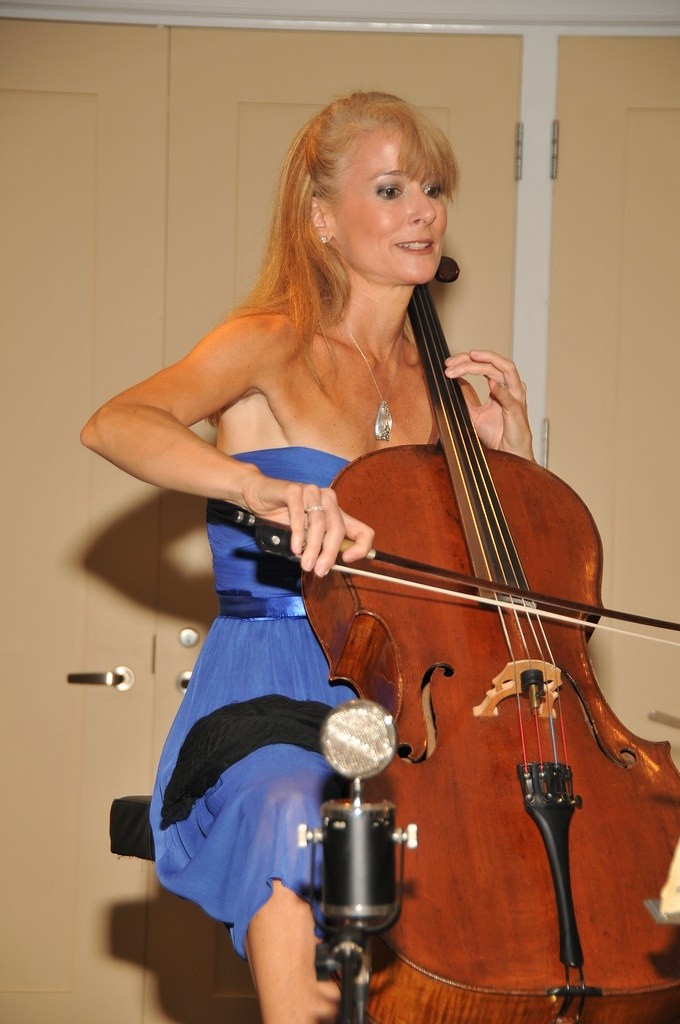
[308, 504, 325, 518]
[499, 381, 505, 386]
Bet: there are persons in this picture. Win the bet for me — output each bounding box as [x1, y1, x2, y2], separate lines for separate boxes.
[82, 87, 534, 1024]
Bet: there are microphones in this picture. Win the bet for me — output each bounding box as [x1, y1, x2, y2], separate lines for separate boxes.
[321, 699, 407, 919]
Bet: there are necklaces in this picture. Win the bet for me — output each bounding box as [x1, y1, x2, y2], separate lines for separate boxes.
[342, 319, 402, 441]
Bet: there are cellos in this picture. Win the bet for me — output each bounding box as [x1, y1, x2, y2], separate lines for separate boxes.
[298, 250, 680, 1024]
